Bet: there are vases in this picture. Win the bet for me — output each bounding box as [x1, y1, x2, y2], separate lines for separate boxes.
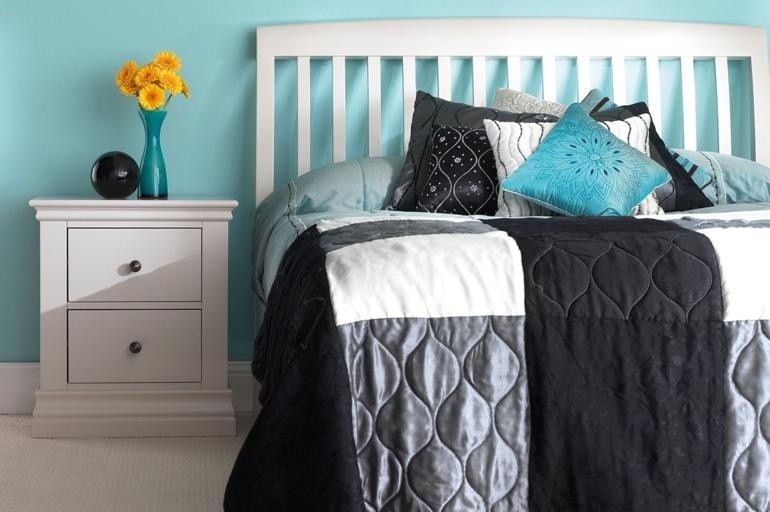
[137, 110, 168, 200]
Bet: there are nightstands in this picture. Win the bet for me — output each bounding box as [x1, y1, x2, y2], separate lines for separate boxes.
[28, 198, 240, 436]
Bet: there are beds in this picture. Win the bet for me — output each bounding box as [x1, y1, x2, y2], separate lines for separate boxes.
[253, 25, 769, 512]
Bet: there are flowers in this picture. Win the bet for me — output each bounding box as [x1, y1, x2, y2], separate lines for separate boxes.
[115, 52, 190, 111]
